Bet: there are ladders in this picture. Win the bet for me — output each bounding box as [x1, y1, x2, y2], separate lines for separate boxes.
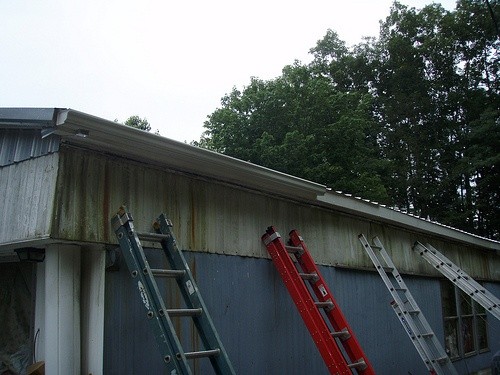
[110, 203, 239, 375]
[262, 224, 379, 375]
[410, 240, 500, 321]
[356, 232, 457, 375]
